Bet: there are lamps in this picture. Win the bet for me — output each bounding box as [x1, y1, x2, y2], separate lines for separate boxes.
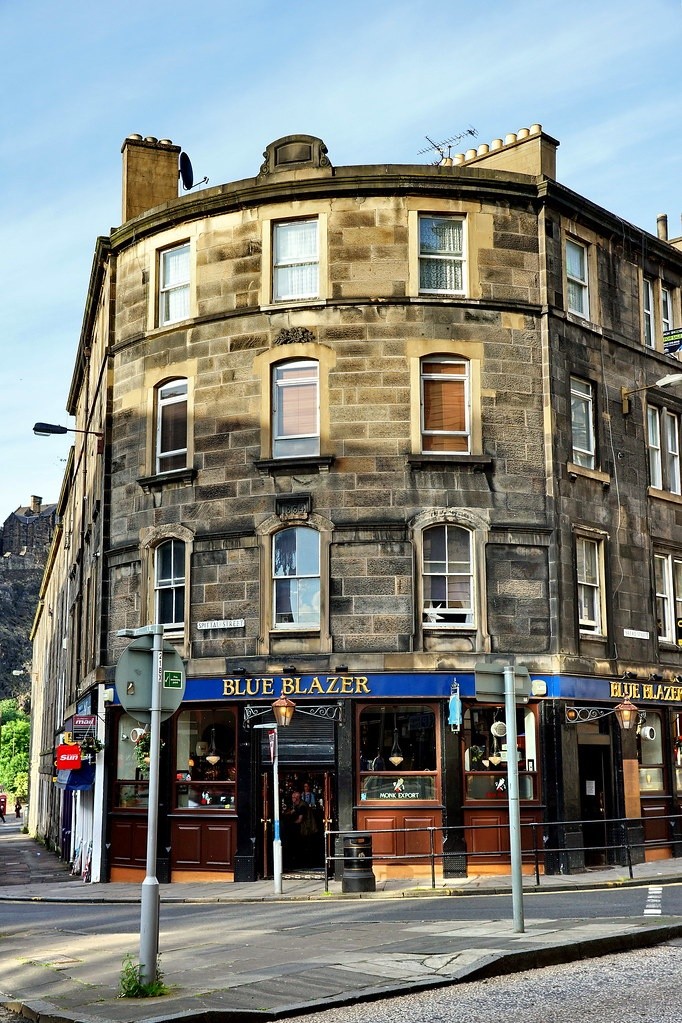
[482, 736, 501, 768]
[336, 664, 348, 672]
[389, 728, 403, 767]
[283, 665, 296, 673]
[13, 670, 39, 675]
[562, 692, 638, 731]
[206, 728, 220, 765]
[623, 671, 682, 683]
[33, 423, 103, 438]
[271, 689, 344, 728]
[233, 667, 246, 675]
[621, 373, 682, 400]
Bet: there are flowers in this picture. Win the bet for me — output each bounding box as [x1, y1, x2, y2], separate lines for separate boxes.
[132, 733, 165, 777]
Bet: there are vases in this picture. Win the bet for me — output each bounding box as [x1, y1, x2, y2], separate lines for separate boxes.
[143, 752, 150, 766]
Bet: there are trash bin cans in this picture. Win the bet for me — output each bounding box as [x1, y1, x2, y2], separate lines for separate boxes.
[338, 830, 376, 894]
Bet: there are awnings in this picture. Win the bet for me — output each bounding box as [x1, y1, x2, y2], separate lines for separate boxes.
[55, 757, 95, 790]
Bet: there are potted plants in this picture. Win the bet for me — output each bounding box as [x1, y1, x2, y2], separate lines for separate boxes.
[79, 736, 106, 756]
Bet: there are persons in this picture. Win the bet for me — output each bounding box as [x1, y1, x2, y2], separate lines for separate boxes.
[15, 801, 22, 818]
[0, 806, 6, 823]
[283, 782, 319, 867]
[360, 753, 364, 771]
[372, 751, 386, 772]
